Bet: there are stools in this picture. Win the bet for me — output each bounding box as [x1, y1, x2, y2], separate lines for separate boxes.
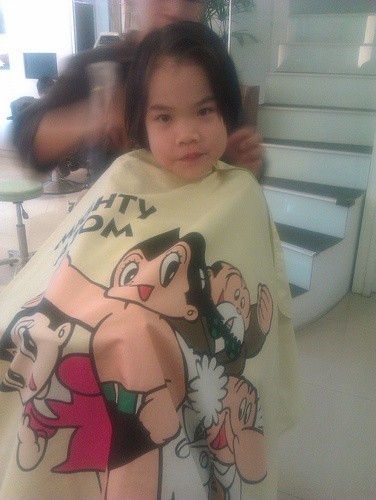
[0, 178, 43, 282]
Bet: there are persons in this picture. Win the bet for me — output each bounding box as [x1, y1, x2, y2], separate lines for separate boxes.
[9, 0, 267, 188]
[36, 77, 56, 98]
[118, 20, 244, 182]
